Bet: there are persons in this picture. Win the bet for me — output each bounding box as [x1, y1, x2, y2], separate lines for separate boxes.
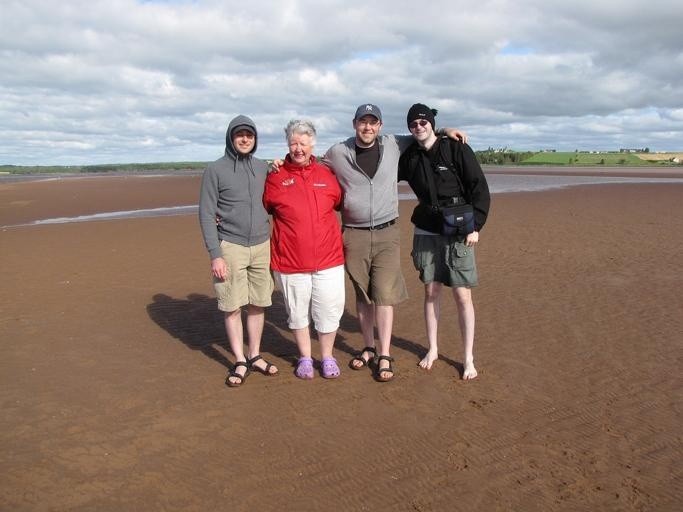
[217, 120, 345, 379]
[271, 103, 468, 382]
[397, 103, 490, 381]
[198, 115, 278, 387]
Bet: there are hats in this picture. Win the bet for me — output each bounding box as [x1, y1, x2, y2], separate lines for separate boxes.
[407, 104, 437, 130]
[355, 104, 383, 121]
[232, 124, 256, 136]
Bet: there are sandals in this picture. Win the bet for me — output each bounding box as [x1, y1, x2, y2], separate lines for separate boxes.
[226, 356, 250, 386]
[320, 357, 340, 379]
[295, 357, 314, 380]
[375, 356, 394, 382]
[247, 356, 279, 376]
[350, 346, 377, 370]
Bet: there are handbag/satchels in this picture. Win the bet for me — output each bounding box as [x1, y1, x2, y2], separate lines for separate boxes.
[439, 205, 475, 236]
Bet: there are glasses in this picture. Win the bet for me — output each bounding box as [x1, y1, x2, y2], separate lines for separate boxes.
[410, 121, 427, 127]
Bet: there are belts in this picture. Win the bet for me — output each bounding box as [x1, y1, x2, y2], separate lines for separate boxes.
[355, 219, 396, 231]
